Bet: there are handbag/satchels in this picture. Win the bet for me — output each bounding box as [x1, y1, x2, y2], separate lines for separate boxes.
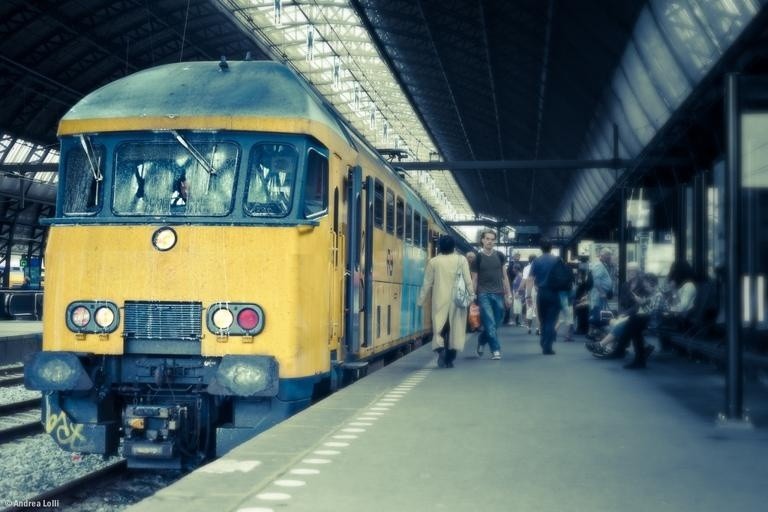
[469, 303, 480, 331]
[549, 260, 575, 291]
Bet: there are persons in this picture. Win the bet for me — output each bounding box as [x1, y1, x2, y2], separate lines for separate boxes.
[525, 236, 563, 354]
[178, 175, 188, 202]
[417, 236, 476, 367]
[466, 227, 611, 334]
[586, 259, 697, 360]
[470, 231, 511, 359]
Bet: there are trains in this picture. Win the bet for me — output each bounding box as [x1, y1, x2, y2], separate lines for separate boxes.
[22, 51, 472, 478]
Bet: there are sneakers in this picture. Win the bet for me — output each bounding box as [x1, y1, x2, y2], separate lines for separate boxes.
[438, 321, 655, 369]
